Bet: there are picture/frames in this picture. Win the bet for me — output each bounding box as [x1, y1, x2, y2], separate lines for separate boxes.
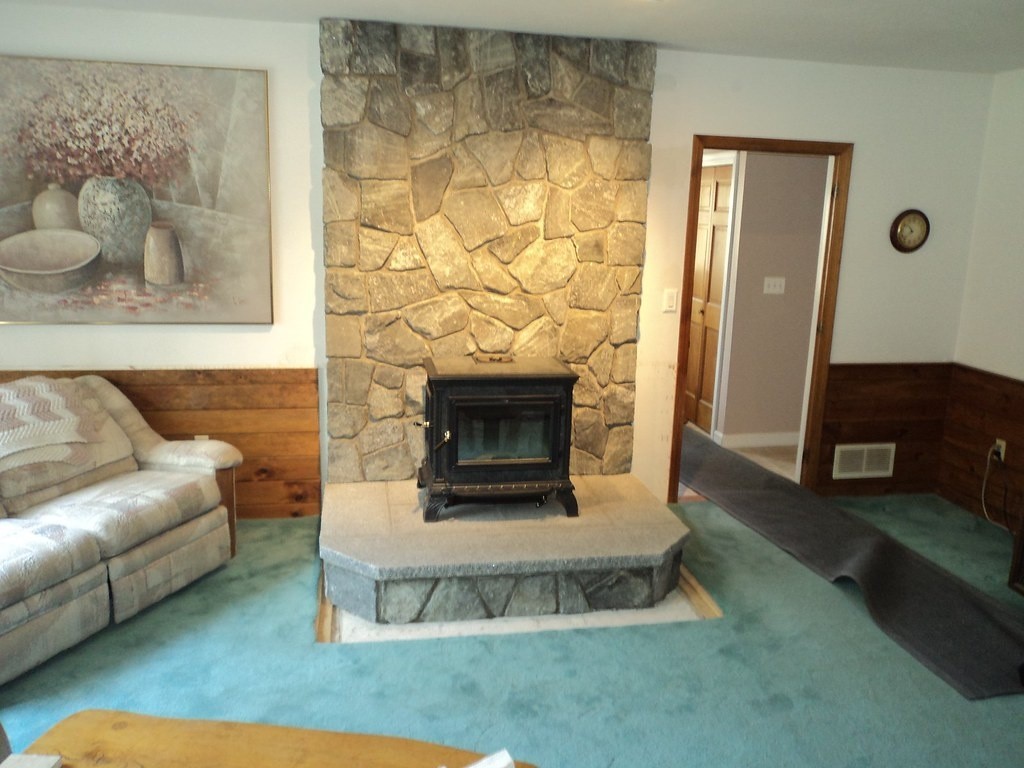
[0, 54, 274, 325]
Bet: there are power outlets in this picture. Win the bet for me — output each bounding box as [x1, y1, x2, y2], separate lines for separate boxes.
[993, 438, 1005, 461]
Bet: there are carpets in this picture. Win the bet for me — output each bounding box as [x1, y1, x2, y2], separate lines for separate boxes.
[679, 426, 1024, 701]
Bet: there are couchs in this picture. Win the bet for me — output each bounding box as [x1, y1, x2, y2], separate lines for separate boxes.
[0, 370, 242, 686]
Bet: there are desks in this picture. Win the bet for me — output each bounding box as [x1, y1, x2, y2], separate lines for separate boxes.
[21, 709, 539, 768]
[417, 357, 579, 522]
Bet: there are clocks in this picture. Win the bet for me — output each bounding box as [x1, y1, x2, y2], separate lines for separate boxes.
[890, 210, 931, 254]
